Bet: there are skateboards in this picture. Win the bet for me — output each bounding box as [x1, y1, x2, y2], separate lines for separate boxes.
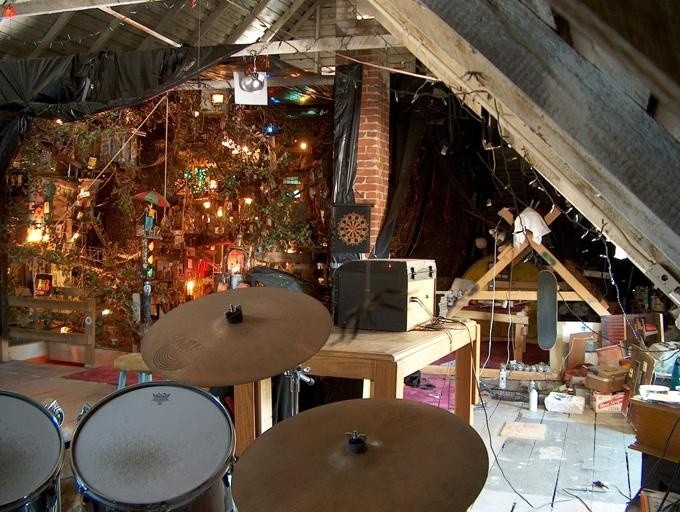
[537, 270, 557, 351]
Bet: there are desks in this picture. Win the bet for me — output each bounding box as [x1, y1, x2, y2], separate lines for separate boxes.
[234, 319, 481, 458]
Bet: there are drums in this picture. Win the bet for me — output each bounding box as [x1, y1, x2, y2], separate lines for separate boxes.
[69, 380, 237, 510]
[1, 389, 65, 510]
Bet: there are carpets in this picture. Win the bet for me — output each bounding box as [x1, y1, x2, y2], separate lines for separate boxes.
[403, 341, 550, 411]
[59, 362, 167, 385]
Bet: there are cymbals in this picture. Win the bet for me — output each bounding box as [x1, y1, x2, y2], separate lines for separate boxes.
[232, 397, 489, 512]
[140, 287, 333, 387]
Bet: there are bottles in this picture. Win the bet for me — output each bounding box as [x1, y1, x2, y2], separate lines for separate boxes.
[499, 364, 507, 391]
[528, 379, 537, 411]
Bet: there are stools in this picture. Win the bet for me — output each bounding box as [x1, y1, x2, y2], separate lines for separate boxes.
[113, 353, 152, 391]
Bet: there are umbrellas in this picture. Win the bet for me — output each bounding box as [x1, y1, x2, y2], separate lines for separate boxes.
[130, 185, 171, 209]
